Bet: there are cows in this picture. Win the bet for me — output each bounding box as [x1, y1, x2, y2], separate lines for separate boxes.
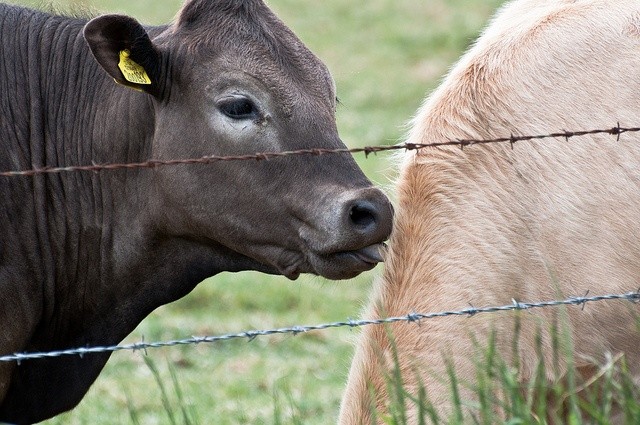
[1, 1, 395, 425]
[337, 0, 639, 425]
